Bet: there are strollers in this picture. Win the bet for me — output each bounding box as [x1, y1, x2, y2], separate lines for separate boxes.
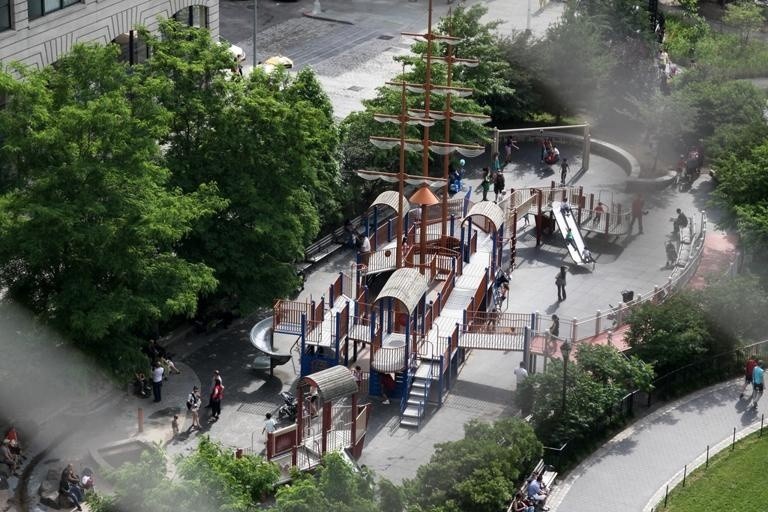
[276, 388, 309, 424]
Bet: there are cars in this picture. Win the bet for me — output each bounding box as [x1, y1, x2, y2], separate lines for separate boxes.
[257, 53, 294, 72]
[218, 32, 245, 66]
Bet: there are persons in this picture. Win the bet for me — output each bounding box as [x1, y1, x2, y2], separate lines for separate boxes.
[512, 471, 551, 512]
[487, 284, 516, 336]
[231, 55, 243, 78]
[670, 209, 688, 234]
[353, 367, 363, 391]
[344, 218, 371, 253]
[740, 354, 757, 400]
[379, 373, 395, 405]
[550, 314, 559, 352]
[262, 413, 277, 444]
[675, 143, 704, 185]
[0, 425, 95, 511]
[447, 135, 604, 263]
[134, 339, 224, 438]
[307, 385, 319, 418]
[415, 204, 422, 228]
[555, 265, 570, 302]
[631, 193, 645, 234]
[514, 362, 529, 391]
[752, 360, 766, 408]
[666, 239, 677, 267]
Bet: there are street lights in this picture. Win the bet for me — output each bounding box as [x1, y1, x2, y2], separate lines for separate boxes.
[558, 338, 573, 440]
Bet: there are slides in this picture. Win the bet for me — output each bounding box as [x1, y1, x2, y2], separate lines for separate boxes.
[552, 200, 595, 272]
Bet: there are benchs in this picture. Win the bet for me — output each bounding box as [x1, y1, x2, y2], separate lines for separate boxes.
[507, 459, 558, 512]
